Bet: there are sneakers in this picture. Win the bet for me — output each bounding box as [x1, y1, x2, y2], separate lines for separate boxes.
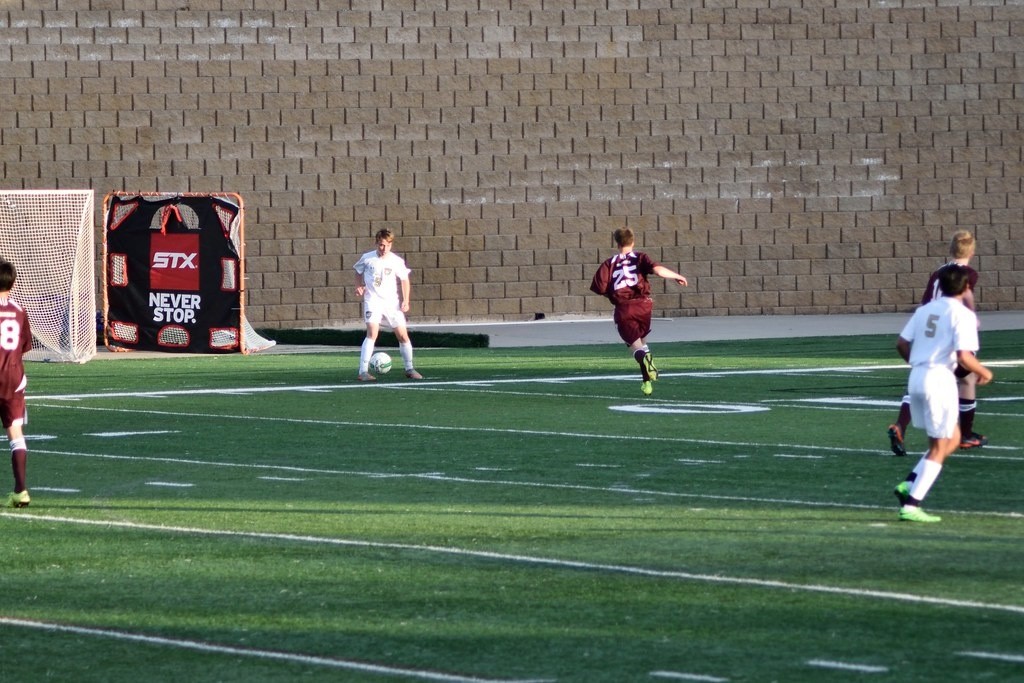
[404, 368, 422, 380]
[898, 504, 942, 522]
[894, 481, 908, 506]
[640, 381, 652, 395]
[5, 490, 30, 508]
[887, 424, 907, 456]
[960, 432, 987, 450]
[643, 353, 659, 383]
[358, 372, 376, 382]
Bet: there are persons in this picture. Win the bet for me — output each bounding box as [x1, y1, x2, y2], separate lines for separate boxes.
[353, 229, 427, 381]
[895, 266, 995, 523]
[589, 227, 688, 397]
[1, 255, 35, 509]
[887, 229, 990, 456]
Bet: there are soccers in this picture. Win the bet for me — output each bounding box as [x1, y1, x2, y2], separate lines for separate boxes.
[370, 352, 392, 374]
